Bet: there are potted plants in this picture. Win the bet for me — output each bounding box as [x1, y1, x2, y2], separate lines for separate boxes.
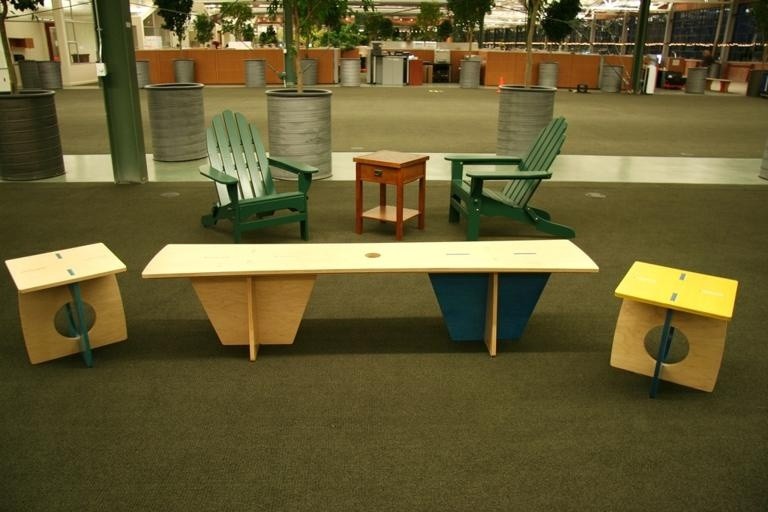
[0, 0, 66, 182]
[444, 0, 495, 89]
[154, 0, 369, 181]
[496, 0, 581, 164]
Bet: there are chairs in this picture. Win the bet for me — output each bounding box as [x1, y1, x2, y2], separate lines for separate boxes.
[197, 109, 318, 244]
[448, 114, 572, 239]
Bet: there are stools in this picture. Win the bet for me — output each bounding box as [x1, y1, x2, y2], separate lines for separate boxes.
[609, 262, 740, 398]
[5, 240, 132, 367]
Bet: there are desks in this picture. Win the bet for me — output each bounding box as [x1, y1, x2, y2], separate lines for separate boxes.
[351, 149, 430, 240]
[141, 236, 599, 362]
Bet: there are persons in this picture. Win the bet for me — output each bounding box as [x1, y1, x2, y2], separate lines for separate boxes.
[700, 49, 717, 69]
